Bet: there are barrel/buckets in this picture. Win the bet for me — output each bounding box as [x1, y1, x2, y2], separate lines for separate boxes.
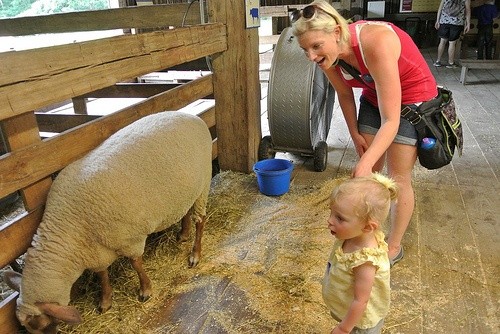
[253, 159, 294, 196]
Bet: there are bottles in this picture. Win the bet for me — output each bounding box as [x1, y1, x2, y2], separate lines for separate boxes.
[426, 18, 429, 24]
[420, 135, 435, 151]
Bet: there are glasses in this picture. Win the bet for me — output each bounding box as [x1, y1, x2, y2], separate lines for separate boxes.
[288, 5, 339, 26]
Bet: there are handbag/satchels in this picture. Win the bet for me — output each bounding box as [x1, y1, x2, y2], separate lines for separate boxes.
[410, 87, 463, 170]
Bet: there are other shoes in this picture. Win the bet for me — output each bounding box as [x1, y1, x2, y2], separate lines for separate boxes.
[433, 61, 441, 67]
[445, 61, 460, 68]
[389, 246, 403, 269]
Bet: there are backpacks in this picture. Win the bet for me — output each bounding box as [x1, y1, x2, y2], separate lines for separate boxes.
[444, 0, 465, 17]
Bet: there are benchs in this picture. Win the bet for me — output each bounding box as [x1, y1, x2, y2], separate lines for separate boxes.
[457, 59, 500, 85]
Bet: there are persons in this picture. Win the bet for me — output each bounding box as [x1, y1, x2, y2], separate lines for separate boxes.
[292, 0, 439, 266]
[322, 174, 400, 334]
[432, 0, 498, 68]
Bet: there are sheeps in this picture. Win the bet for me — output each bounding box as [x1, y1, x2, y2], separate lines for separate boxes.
[0, 110, 213, 334]
